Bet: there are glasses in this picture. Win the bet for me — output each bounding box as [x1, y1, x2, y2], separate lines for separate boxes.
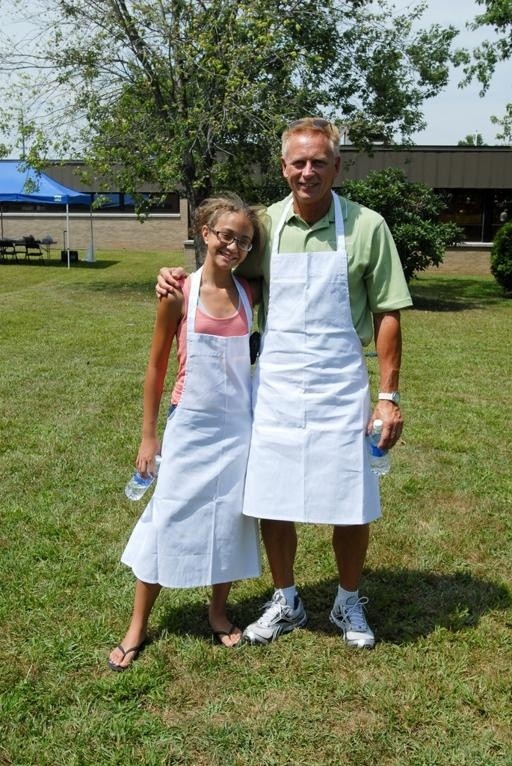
[205, 222, 253, 251]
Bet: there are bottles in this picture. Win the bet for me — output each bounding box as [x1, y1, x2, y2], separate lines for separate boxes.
[369, 418, 390, 476]
[123, 454, 163, 501]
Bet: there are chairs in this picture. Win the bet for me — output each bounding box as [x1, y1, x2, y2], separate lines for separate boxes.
[1, 238, 48, 266]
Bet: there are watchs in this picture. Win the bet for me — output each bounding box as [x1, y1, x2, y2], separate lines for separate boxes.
[377, 391, 400, 404]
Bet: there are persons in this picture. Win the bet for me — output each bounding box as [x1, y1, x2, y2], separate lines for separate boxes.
[155, 117, 413, 649]
[106, 192, 272, 673]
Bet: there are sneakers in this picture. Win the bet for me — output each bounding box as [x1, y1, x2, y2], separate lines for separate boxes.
[241, 591, 308, 645]
[329, 595, 376, 649]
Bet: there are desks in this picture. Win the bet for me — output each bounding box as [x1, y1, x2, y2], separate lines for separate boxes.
[7, 240, 58, 260]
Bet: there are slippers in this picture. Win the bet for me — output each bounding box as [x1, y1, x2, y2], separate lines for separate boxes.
[107, 644, 139, 670]
[215, 624, 236, 640]
